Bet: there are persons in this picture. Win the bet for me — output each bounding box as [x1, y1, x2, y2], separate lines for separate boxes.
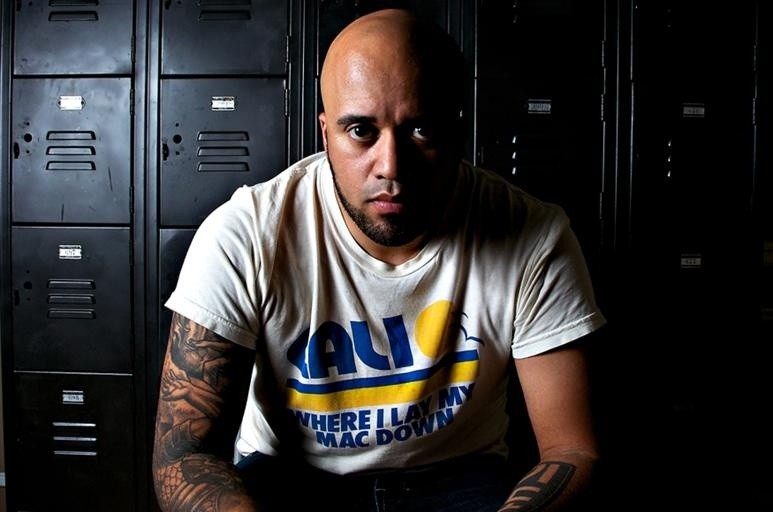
[152, 8, 608, 512]
